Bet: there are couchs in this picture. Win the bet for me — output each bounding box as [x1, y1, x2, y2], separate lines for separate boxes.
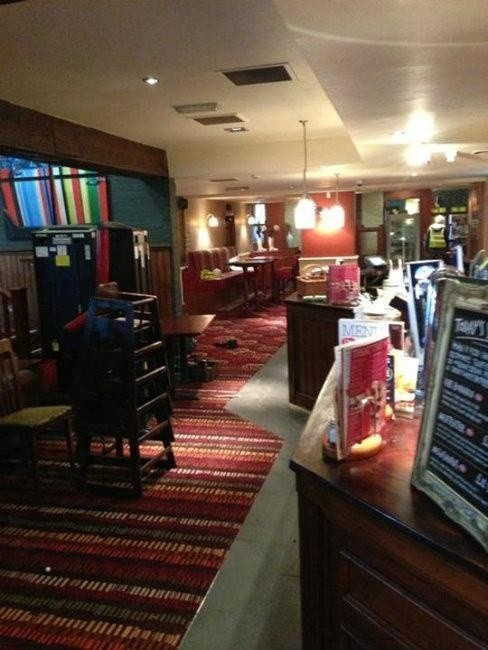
[185, 246, 300, 311]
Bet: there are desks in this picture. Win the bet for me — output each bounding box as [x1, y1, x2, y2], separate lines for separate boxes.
[226, 255, 280, 318]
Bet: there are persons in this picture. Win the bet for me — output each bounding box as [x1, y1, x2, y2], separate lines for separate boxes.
[425, 215, 450, 260]
[452, 214, 469, 256]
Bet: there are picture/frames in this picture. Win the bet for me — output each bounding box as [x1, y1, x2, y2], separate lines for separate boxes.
[411, 278, 487, 551]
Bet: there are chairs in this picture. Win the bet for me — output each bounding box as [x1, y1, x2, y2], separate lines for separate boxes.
[0, 339, 75, 493]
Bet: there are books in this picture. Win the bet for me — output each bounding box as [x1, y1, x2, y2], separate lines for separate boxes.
[334, 317, 405, 460]
[328, 264, 361, 306]
